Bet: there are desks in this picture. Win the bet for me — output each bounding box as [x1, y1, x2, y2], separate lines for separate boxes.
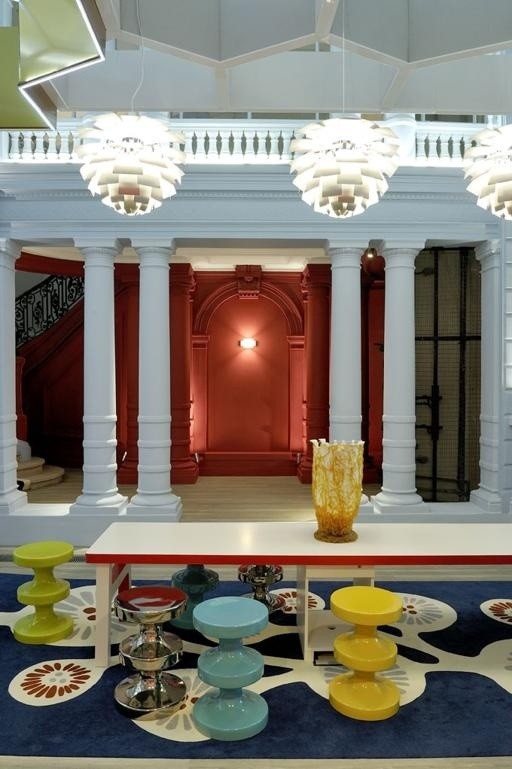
[86, 518, 512, 666]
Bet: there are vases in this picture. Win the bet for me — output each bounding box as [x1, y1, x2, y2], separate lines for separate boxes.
[308, 437, 366, 544]
